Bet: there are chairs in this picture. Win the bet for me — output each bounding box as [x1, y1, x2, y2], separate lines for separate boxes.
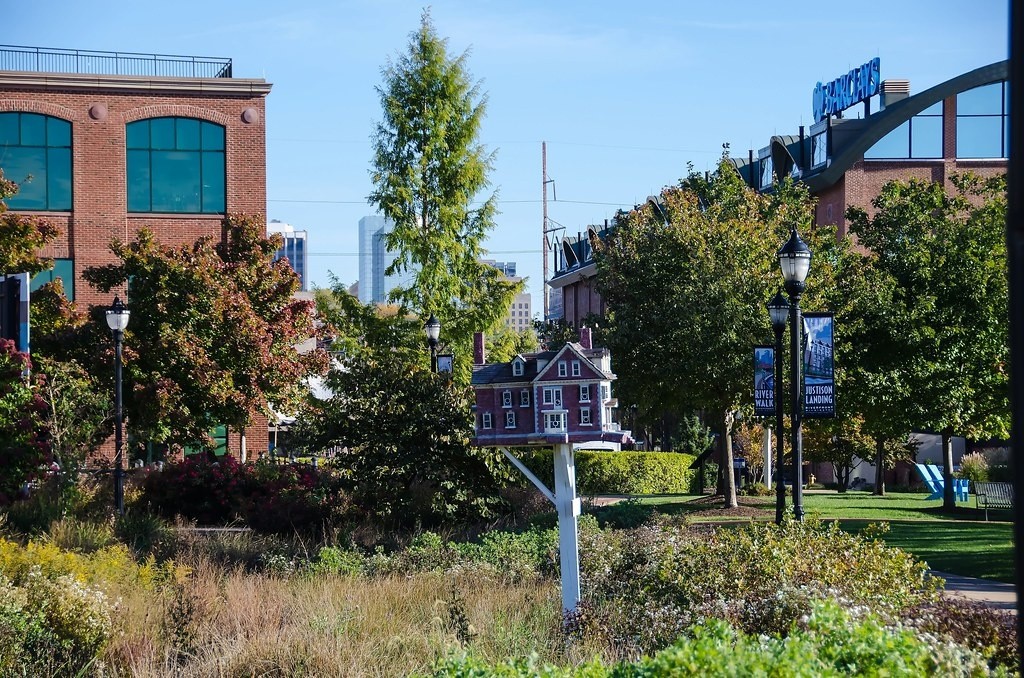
[914, 464, 969, 502]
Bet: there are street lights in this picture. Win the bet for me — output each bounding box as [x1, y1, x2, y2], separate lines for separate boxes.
[105, 294, 133, 518]
[777, 229, 811, 522]
[764, 287, 790, 525]
[424, 312, 439, 373]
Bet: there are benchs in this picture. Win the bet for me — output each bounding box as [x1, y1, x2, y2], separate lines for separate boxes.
[974, 481, 1016, 521]
